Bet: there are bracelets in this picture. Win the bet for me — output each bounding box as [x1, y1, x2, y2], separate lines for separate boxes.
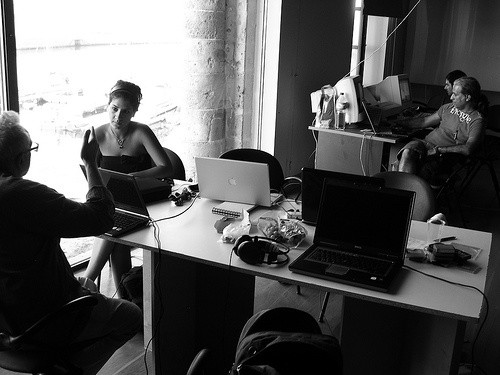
[434, 146, 439, 150]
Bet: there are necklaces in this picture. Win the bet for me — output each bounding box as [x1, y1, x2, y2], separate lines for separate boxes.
[453, 112, 475, 140]
[110, 122, 132, 149]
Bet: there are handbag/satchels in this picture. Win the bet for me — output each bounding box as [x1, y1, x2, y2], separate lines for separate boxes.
[123, 264, 143, 308]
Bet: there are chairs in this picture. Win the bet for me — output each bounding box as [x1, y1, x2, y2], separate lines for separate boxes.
[318, 105, 500, 324]
[0, 295, 107, 375]
[219, 148, 284, 192]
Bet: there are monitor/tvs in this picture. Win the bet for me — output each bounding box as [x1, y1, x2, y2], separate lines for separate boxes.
[310, 75, 365, 128]
[376, 73, 413, 107]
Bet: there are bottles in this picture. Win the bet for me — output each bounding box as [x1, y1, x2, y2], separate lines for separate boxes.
[334, 93, 347, 130]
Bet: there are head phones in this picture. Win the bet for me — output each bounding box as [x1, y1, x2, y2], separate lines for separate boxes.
[233, 234, 290, 265]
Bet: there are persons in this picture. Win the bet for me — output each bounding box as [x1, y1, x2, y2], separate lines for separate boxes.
[0, 110, 142, 375]
[395, 70, 484, 174]
[83, 80, 175, 301]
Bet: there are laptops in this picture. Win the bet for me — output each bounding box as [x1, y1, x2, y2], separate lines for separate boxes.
[301, 166, 352, 224]
[194, 156, 283, 207]
[80, 164, 150, 237]
[287, 175, 416, 292]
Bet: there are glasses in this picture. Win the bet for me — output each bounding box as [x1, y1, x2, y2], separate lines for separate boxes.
[22, 142, 39, 154]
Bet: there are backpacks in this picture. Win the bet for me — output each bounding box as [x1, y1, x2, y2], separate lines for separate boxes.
[229, 306, 341, 375]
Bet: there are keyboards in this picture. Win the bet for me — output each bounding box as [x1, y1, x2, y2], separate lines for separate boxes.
[377, 127, 392, 134]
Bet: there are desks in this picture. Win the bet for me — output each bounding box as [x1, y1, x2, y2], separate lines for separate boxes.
[307, 125, 419, 176]
[96, 178, 492, 375]
